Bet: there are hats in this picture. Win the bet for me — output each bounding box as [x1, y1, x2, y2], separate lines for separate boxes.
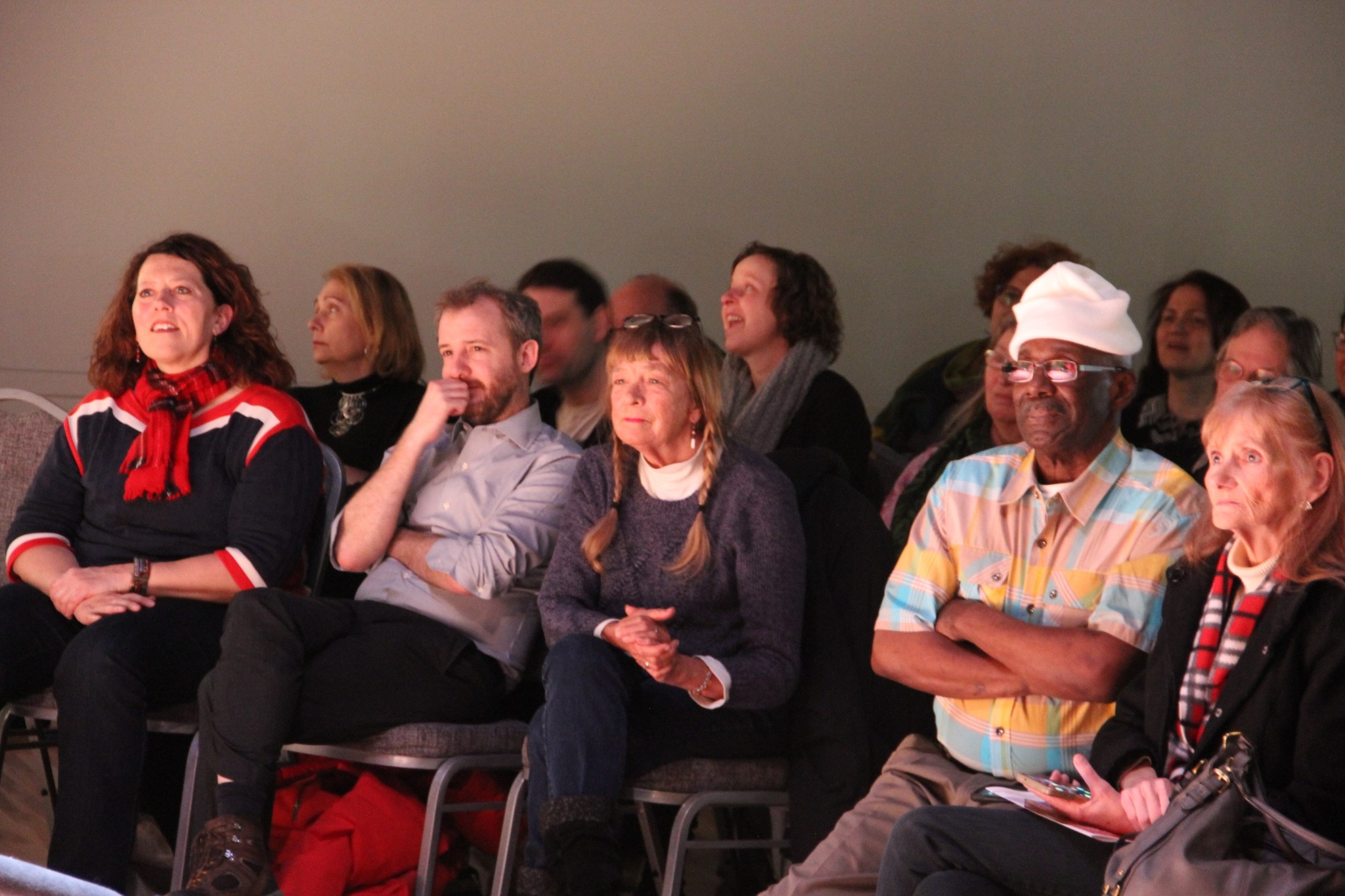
[1012, 258, 1143, 358]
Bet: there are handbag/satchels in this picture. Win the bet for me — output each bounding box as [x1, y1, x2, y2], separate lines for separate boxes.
[1102, 734, 1344, 896]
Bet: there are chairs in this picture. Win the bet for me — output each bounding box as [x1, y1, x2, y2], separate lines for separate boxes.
[1, 391, 792, 895]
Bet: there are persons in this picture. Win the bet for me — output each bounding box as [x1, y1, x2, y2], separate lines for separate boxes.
[1211, 306, 1322, 406]
[749, 259, 1226, 896]
[166, 275, 585, 896]
[514, 257, 614, 447]
[871, 239, 1095, 544]
[525, 312, 807, 896]
[608, 274, 699, 344]
[874, 375, 1345, 896]
[889, 315, 1023, 547]
[0, 229, 327, 896]
[1120, 268, 1250, 490]
[1329, 311, 1345, 411]
[132, 264, 428, 896]
[717, 237, 886, 506]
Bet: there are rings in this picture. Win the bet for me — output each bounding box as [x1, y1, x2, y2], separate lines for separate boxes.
[645, 661, 650, 668]
[655, 629, 660, 633]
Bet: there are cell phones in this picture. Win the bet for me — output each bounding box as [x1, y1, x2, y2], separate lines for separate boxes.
[1015, 772, 1091, 802]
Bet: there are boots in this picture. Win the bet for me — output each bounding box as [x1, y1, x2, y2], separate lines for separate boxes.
[540, 796, 621, 896]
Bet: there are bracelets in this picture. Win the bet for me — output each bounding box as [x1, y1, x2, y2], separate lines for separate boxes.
[131, 557, 150, 595]
[692, 669, 712, 695]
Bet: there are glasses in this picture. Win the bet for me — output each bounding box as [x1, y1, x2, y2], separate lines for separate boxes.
[1214, 359, 1282, 390]
[1261, 373, 1333, 457]
[623, 311, 708, 350]
[1000, 359, 1128, 384]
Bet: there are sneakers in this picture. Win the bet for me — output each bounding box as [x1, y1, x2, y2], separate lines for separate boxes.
[164, 815, 278, 896]
